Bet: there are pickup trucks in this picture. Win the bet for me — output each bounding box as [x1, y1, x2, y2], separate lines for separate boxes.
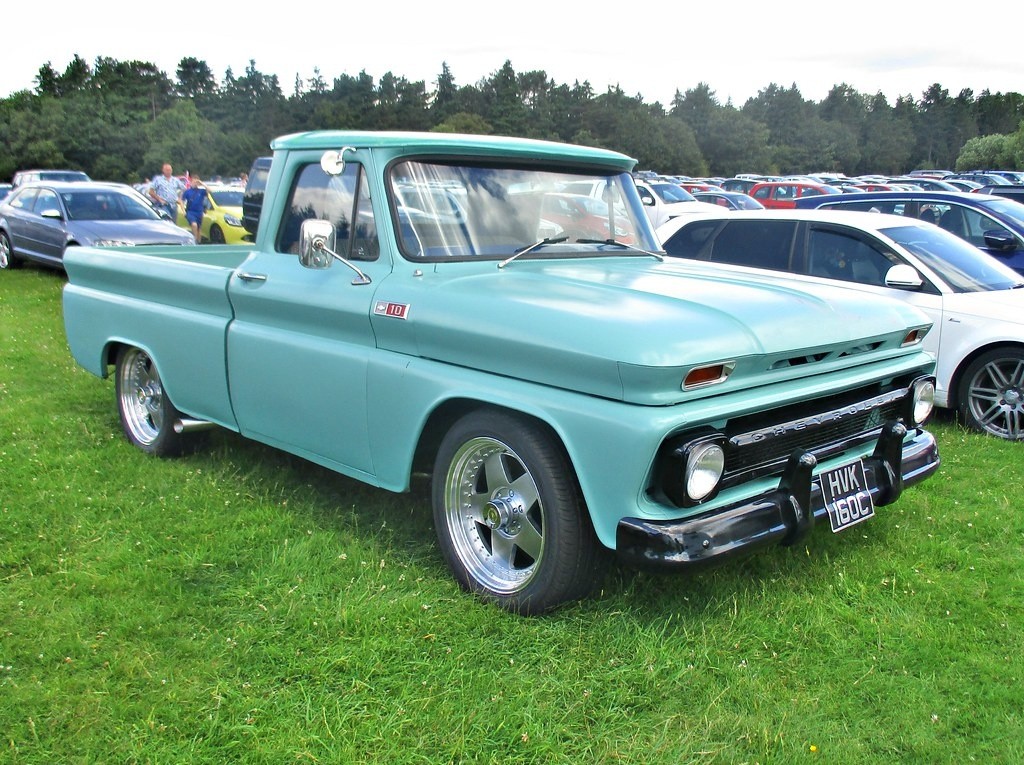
[61, 128, 940, 620]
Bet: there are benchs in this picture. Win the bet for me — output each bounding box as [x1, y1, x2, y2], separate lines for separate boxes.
[289, 233, 525, 262]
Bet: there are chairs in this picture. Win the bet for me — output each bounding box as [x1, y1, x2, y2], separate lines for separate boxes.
[919, 208, 936, 223]
[938, 208, 955, 232]
[850, 238, 880, 283]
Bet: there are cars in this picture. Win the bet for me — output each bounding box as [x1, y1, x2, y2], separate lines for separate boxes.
[178, 184, 256, 245]
[651, 206, 1024, 442]
[0, 179, 196, 275]
[582, 169, 1024, 289]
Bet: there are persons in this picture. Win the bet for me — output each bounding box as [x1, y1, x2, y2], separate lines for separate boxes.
[148, 163, 187, 224]
[180, 175, 210, 244]
[146, 172, 249, 189]
